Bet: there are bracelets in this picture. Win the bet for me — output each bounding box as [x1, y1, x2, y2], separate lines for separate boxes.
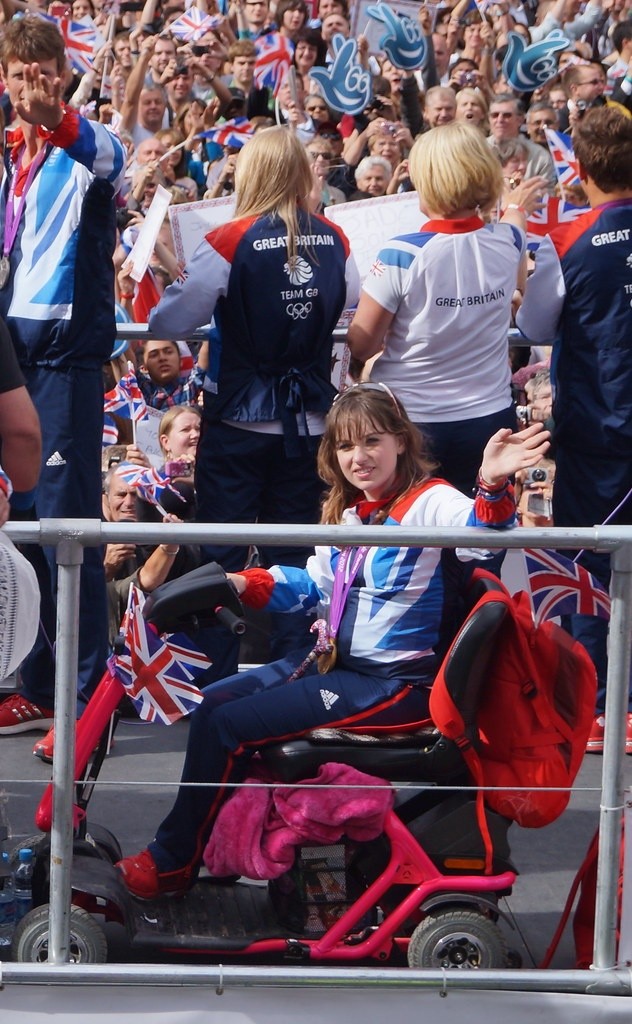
[161, 545, 179, 554]
[471, 476, 510, 493]
[506, 204, 526, 218]
[478, 469, 506, 486]
[90, 66, 99, 74]
[207, 73, 216, 82]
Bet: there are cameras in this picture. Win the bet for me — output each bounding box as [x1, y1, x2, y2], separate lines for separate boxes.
[465, 74, 476, 83]
[381, 123, 401, 134]
[522, 468, 550, 483]
[175, 66, 191, 74]
[191, 43, 212, 56]
[516, 406, 533, 420]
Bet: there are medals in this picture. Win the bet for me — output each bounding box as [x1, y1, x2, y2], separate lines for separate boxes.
[0, 258, 10, 288]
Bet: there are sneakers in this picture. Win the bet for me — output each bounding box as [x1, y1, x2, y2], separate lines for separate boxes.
[0, 693, 53, 734]
[114, 849, 192, 903]
[31, 724, 55, 765]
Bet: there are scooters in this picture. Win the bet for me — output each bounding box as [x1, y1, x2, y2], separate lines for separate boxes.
[3, 558, 524, 969]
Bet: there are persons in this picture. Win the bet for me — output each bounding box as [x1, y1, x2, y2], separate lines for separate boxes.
[113, 388, 551, 899]
[0, 0, 632, 760]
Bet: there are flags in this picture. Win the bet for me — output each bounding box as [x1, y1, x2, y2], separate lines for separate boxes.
[104, 369, 149, 421]
[36, 12, 96, 71]
[117, 463, 170, 502]
[254, 35, 295, 96]
[200, 116, 255, 148]
[131, 587, 212, 680]
[168, 9, 216, 40]
[115, 612, 205, 724]
[546, 130, 580, 186]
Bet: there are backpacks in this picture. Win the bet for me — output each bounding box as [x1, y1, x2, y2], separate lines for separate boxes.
[429, 567, 599, 830]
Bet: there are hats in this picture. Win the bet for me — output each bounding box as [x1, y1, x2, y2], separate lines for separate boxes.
[228, 88, 245, 102]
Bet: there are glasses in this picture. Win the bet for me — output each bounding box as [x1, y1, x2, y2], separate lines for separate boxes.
[488, 111, 519, 119]
[331, 382, 401, 418]
[577, 79, 598, 87]
[307, 104, 328, 111]
[311, 152, 332, 160]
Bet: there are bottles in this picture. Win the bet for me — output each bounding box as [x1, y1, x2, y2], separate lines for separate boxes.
[0, 855, 16, 945]
[14, 848, 34, 926]
